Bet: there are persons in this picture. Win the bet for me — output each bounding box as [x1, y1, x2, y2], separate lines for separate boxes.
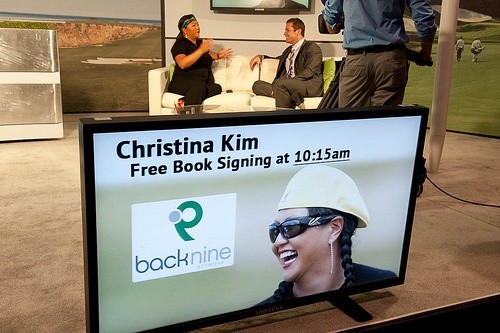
[456, 36, 465, 61]
[323, 0, 438, 108]
[249, 18, 324, 108]
[250, 165, 399, 312]
[168, 14, 233, 113]
[471, 37, 483, 62]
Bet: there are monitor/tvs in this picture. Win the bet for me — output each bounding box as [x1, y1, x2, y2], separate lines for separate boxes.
[78, 104, 428, 333]
[209, 0, 316, 15]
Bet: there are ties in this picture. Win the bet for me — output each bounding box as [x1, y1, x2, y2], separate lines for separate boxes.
[286, 49, 296, 78]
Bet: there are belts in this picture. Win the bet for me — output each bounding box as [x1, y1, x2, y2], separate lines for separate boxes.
[347, 43, 404, 54]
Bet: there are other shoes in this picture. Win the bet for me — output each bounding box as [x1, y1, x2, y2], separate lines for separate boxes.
[174, 100, 185, 112]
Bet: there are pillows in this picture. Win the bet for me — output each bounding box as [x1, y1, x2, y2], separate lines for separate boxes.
[320, 57, 336, 94]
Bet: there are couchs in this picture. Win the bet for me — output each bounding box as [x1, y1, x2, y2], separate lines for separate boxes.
[148, 55, 345, 116]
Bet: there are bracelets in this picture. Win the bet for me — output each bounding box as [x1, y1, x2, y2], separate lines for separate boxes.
[217, 53, 221, 59]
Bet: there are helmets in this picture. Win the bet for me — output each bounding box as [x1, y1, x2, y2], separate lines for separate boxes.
[278, 163, 369, 228]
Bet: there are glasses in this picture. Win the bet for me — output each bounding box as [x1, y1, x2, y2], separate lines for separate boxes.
[268, 216, 334, 242]
